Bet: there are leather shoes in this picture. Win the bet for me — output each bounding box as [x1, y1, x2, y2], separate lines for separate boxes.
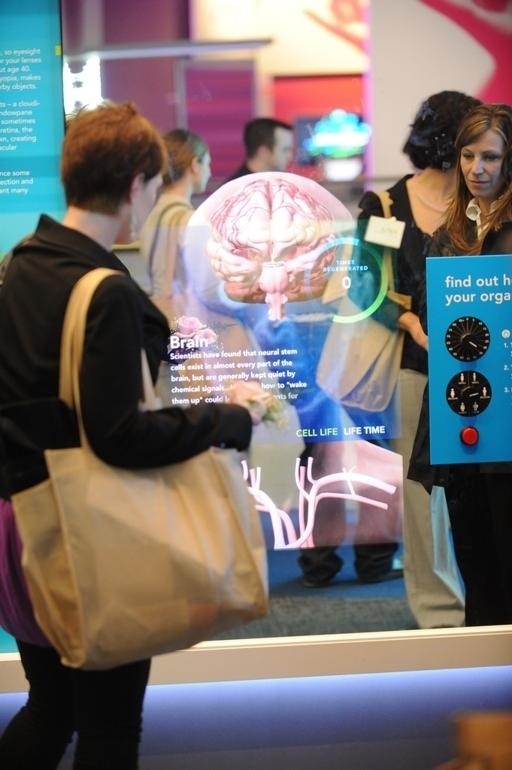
[305, 555, 404, 587]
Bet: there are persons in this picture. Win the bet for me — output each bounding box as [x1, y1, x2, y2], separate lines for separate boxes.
[1, 103, 404, 770]
[345, 91, 468, 629]
[407, 103, 512, 630]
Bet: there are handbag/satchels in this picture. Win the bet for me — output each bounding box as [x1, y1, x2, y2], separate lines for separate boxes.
[315, 293, 406, 412]
[9, 444, 270, 670]
[148, 293, 256, 386]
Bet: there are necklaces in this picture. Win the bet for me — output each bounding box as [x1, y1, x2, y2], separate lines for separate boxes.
[407, 174, 454, 215]
